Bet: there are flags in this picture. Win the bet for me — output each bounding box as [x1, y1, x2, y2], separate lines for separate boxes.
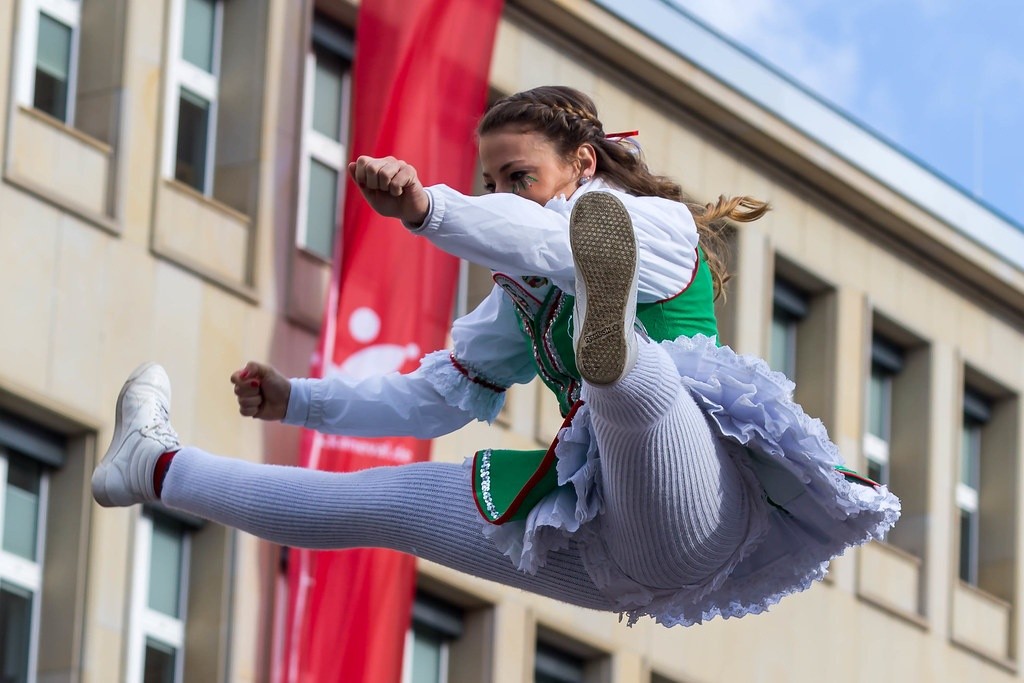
[269, 0, 504, 683]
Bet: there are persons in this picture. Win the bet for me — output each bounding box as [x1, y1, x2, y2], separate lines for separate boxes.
[95, 85, 902, 628]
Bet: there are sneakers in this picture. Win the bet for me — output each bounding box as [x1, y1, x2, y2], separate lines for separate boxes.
[90, 360, 182, 508]
[568, 188, 638, 390]
